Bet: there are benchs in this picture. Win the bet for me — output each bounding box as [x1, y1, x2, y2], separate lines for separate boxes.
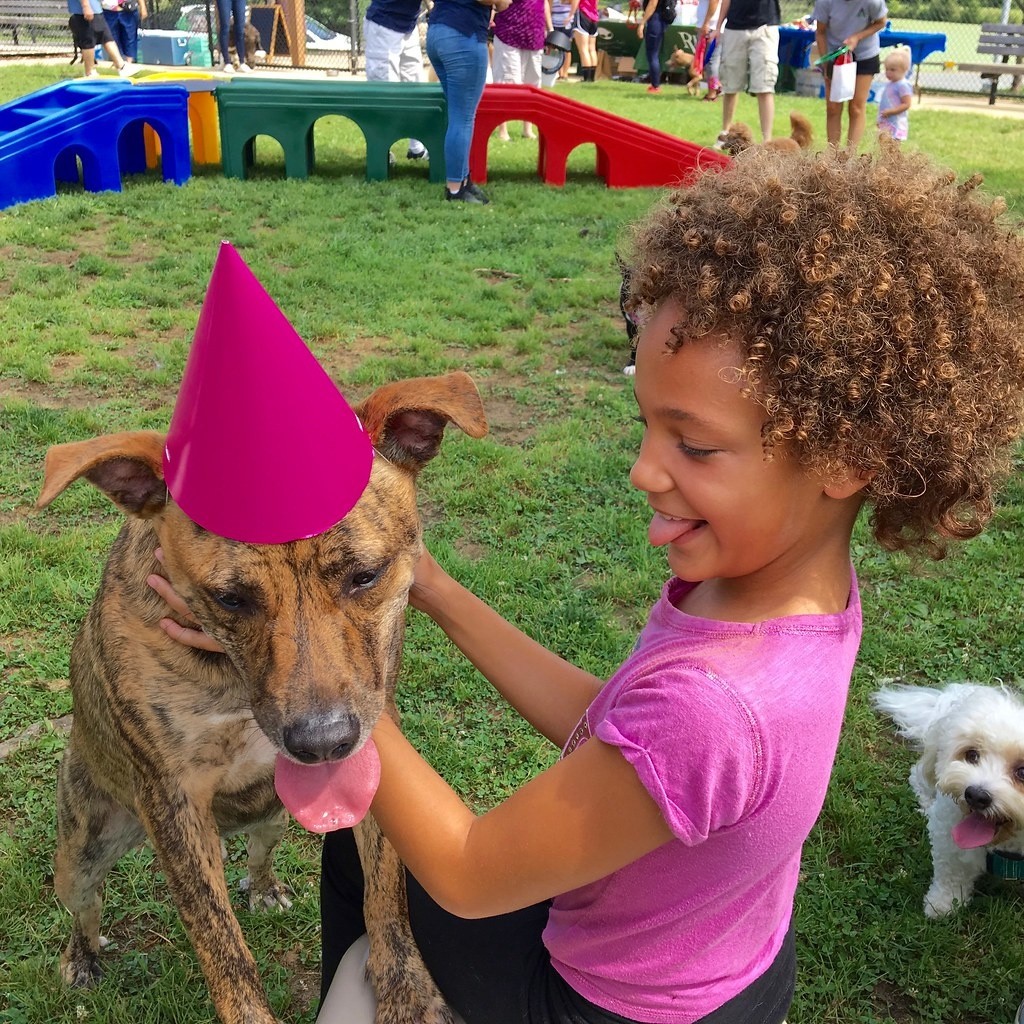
[958, 23, 1024, 105]
[0, 0, 70, 46]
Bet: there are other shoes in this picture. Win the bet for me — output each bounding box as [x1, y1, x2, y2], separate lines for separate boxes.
[110, 64, 117, 69]
[238, 63, 254, 73]
[647, 86, 659, 93]
[88, 69, 100, 77]
[406, 147, 430, 161]
[462, 179, 489, 204]
[119, 61, 142, 78]
[702, 85, 721, 103]
[446, 180, 480, 204]
[223, 63, 236, 73]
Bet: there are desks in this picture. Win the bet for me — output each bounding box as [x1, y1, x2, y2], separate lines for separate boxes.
[571, 21, 699, 84]
[779, 28, 946, 104]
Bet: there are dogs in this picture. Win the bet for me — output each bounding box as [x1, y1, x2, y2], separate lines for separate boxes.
[868, 678, 1024, 920]
[665, 43, 724, 97]
[35, 371, 494, 1024]
[613, 251, 656, 375]
[716, 109, 815, 161]
[212, 13, 264, 70]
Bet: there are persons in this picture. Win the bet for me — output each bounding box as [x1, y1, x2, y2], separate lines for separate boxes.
[67, 0, 148, 78]
[877, 46, 912, 144]
[425, 0, 513, 204]
[363, 0, 434, 163]
[811, 0, 888, 148]
[487, 0, 607, 141]
[635, 0, 670, 93]
[695, 0, 782, 148]
[144, 139, 1024, 1024]
[216, 0, 252, 73]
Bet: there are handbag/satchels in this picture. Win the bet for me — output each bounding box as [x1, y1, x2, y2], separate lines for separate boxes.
[829, 48, 858, 102]
[122, 0, 138, 12]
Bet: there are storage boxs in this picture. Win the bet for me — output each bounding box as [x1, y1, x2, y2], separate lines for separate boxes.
[797, 69, 826, 97]
[140, 29, 217, 67]
[618, 56, 638, 77]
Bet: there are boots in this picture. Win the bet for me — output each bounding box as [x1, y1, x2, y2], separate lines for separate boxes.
[581, 65, 597, 82]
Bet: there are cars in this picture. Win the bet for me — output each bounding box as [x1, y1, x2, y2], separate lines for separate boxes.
[173, 3, 359, 53]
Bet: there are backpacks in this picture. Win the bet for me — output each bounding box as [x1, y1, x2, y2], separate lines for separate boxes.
[657, 0, 676, 25]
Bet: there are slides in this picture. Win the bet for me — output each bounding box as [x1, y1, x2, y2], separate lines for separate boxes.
[470, 80, 744, 189]
